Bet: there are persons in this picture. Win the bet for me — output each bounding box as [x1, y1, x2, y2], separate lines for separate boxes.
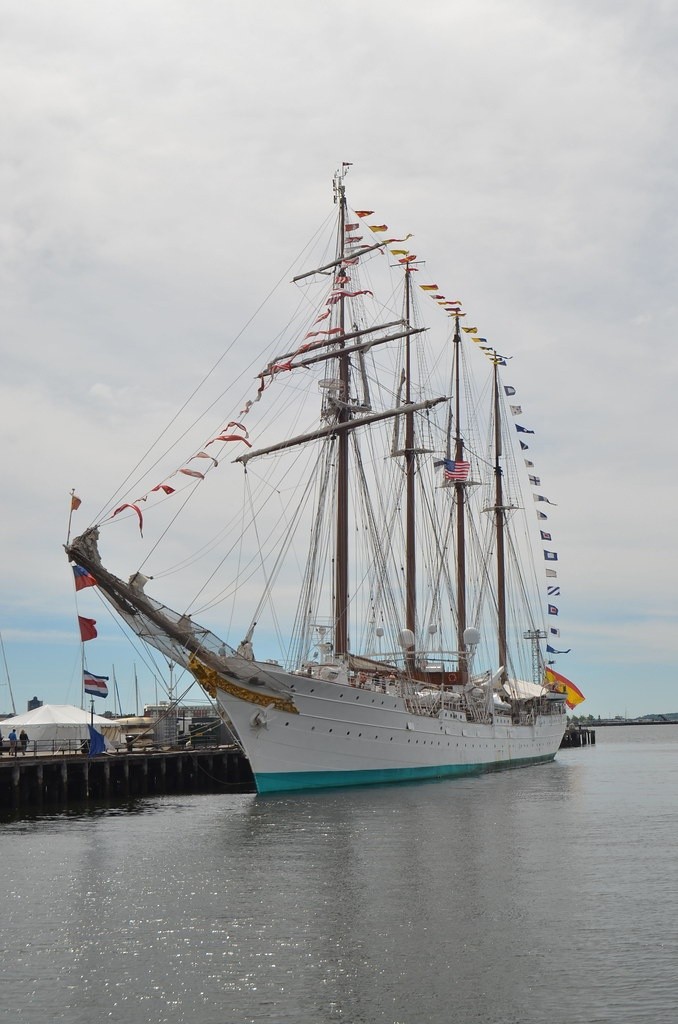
[578, 717, 584, 729]
[357, 669, 396, 688]
[7, 729, 17, 755]
[19, 728, 29, 756]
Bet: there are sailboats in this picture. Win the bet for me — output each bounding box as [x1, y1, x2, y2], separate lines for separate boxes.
[60, 160, 569, 798]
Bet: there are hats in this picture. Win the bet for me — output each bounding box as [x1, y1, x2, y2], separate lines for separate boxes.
[13, 729, 15, 731]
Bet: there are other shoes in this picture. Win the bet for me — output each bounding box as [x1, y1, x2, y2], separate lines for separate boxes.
[1, 753, 4, 755]
[22, 753, 25, 755]
[8, 752, 10, 756]
[11, 754, 14, 756]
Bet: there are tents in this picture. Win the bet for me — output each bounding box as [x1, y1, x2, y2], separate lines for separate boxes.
[0, 704, 123, 751]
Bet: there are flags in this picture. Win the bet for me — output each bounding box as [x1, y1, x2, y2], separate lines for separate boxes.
[443, 458, 469, 480]
[355, 209, 502, 363]
[82, 671, 109, 698]
[113, 223, 384, 536]
[78, 617, 98, 641]
[72, 562, 97, 590]
[504, 385, 585, 710]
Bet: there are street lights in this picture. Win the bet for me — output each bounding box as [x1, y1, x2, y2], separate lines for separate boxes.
[167, 662, 174, 751]
